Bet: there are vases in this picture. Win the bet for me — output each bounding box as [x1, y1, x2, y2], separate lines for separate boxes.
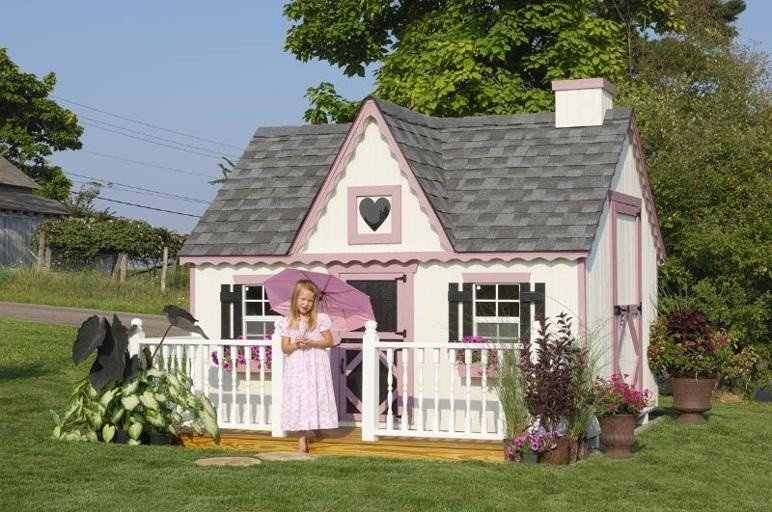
[459, 362, 495, 380]
[665, 378, 717, 428]
[596, 414, 638, 461]
[233, 361, 266, 372]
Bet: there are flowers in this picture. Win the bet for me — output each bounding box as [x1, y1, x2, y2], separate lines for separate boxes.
[211, 333, 278, 371]
[646, 276, 736, 379]
[590, 371, 657, 414]
[455, 335, 498, 365]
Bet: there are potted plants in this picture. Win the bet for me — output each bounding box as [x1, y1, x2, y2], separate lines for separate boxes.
[479, 288, 623, 466]
[48, 304, 222, 447]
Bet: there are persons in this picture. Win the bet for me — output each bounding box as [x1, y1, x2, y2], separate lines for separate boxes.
[280, 279, 338, 456]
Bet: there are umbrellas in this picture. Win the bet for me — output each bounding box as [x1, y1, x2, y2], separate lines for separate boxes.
[264, 267, 377, 341]
